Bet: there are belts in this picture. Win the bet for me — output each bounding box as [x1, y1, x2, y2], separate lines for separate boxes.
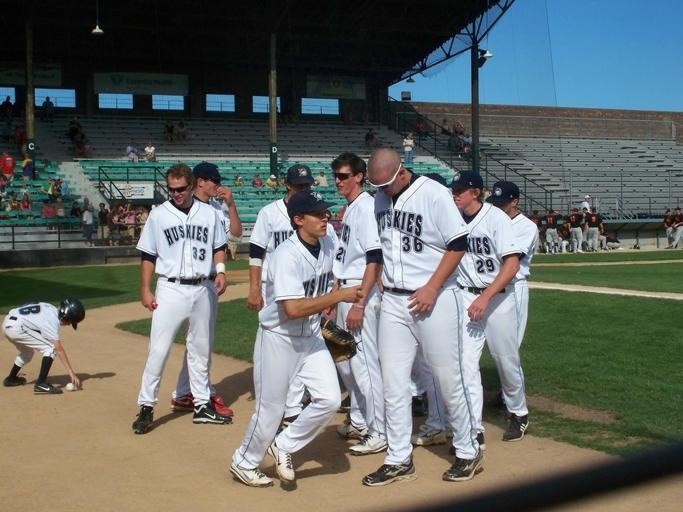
[167, 278, 207, 284]
[383, 285, 418, 295]
[459, 284, 506, 293]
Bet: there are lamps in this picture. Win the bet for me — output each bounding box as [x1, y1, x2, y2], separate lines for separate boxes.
[481, 0, 493, 60]
[404, 65, 416, 84]
[90, 0, 103, 35]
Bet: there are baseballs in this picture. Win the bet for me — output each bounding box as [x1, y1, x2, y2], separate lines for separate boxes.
[67, 383, 75, 392]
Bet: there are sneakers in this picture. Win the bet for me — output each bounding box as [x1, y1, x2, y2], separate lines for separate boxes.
[442, 456, 484, 484]
[3, 375, 29, 387]
[338, 395, 488, 455]
[544, 245, 610, 254]
[362, 461, 418, 486]
[34, 380, 63, 395]
[665, 243, 680, 249]
[265, 438, 303, 481]
[230, 459, 272, 487]
[81, 239, 139, 246]
[170, 393, 235, 423]
[132, 406, 155, 434]
[502, 413, 530, 442]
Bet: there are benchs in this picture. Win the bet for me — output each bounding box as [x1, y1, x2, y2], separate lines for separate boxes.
[412, 135, 682, 227]
[1, 107, 466, 253]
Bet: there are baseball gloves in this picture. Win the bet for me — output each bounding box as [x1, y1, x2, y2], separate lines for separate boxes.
[321, 316, 357, 362]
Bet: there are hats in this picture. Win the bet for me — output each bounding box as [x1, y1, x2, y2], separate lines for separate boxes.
[287, 190, 338, 214]
[665, 207, 681, 213]
[448, 171, 484, 191]
[533, 207, 596, 213]
[193, 163, 230, 181]
[584, 194, 591, 199]
[270, 175, 277, 178]
[287, 164, 314, 186]
[486, 182, 520, 203]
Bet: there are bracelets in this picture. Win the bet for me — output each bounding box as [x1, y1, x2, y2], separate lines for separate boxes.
[352, 303, 363, 310]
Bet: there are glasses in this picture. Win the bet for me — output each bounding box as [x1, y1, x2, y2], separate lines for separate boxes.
[368, 163, 403, 190]
[329, 171, 351, 180]
[165, 182, 189, 191]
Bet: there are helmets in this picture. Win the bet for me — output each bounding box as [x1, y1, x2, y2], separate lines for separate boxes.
[62, 297, 85, 328]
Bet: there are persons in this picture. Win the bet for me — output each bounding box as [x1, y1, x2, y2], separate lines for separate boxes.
[446, 170, 529, 457]
[529, 195, 682, 256]
[229, 191, 363, 487]
[248, 164, 340, 432]
[228, 169, 345, 236]
[362, 147, 486, 486]
[130, 164, 230, 434]
[2, 298, 85, 396]
[337, 106, 483, 168]
[329, 151, 388, 454]
[0, 97, 187, 252]
[484, 178, 538, 413]
[166, 163, 243, 419]
